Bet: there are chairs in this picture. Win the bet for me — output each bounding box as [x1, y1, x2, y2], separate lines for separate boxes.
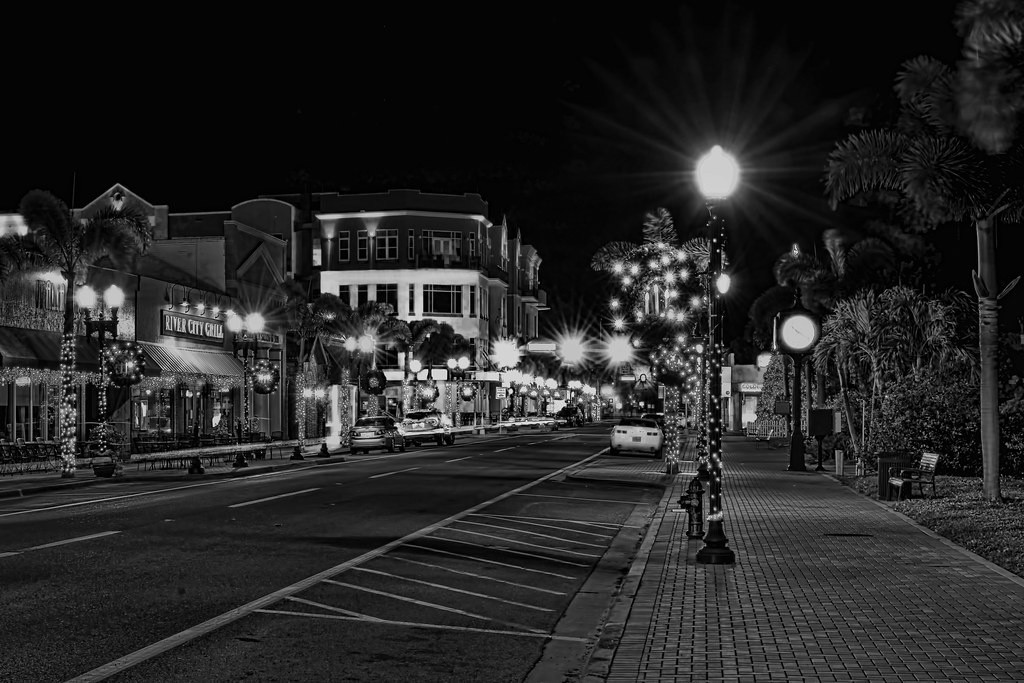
[0, 429, 283, 476]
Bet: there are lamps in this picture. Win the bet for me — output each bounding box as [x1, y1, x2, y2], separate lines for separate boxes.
[168, 281, 231, 318]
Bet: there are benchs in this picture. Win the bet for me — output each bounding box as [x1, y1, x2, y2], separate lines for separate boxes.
[887, 452, 940, 501]
[755, 429, 773, 449]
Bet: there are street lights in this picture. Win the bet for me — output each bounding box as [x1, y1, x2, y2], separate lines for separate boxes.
[76, 283, 126, 454]
[694, 143, 741, 567]
[448, 355, 472, 430]
[227, 313, 266, 444]
[343, 336, 375, 417]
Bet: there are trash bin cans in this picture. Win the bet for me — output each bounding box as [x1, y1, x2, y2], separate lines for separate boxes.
[874, 451, 913, 500]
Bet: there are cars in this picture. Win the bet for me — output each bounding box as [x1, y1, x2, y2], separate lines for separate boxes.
[555, 406, 585, 428]
[400, 409, 456, 446]
[349, 416, 407, 453]
[611, 411, 667, 459]
